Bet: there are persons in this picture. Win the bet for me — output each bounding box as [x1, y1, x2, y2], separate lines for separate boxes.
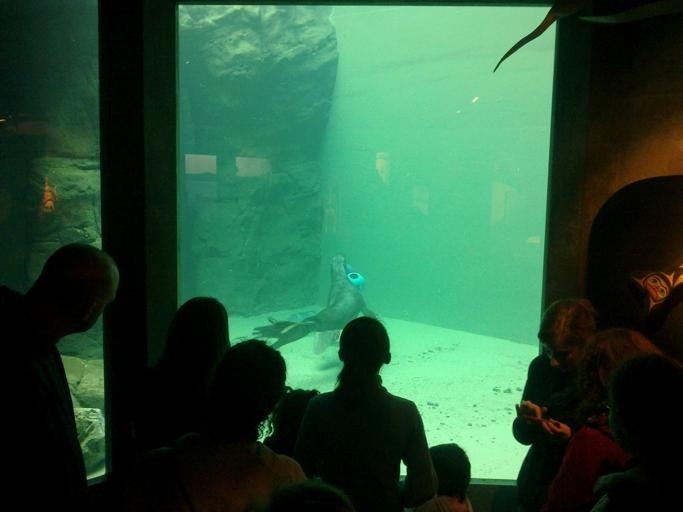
[534, 323, 659, 511]
[571, 173, 683, 361]
[263, 388, 320, 452]
[412, 444, 477, 512]
[129, 336, 310, 512]
[0, 240, 120, 512]
[589, 351, 681, 510]
[109, 294, 239, 478]
[510, 294, 606, 511]
[288, 315, 437, 511]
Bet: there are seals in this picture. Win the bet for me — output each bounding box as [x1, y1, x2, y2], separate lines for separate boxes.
[249, 254, 377, 350]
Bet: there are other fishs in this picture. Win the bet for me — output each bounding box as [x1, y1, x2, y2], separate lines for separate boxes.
[39, 175, 56, 214]
